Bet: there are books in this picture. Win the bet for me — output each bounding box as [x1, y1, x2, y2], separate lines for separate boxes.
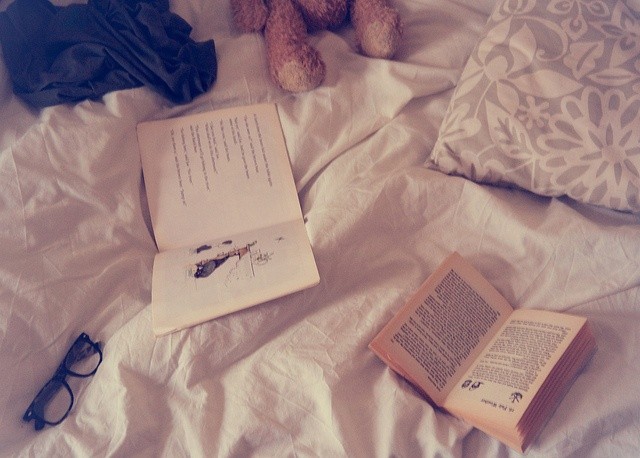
[136, 100, 321, 336]
[369, 249, 598, 452]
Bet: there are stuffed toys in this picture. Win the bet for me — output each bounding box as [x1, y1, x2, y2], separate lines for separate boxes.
[229, 0, 405, 93]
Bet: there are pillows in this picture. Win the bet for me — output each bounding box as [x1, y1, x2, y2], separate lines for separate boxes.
[422, 0, 640, 214]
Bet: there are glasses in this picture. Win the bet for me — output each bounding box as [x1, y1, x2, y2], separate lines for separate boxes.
[23, 332, 102, 431]
